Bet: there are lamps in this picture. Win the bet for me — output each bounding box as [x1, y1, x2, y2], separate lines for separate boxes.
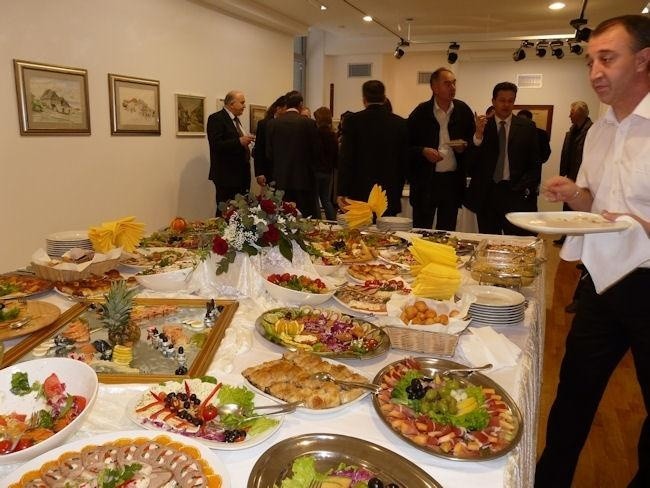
[447, 42, 460, 64]
[569, 18, 592, 43]
[394, 38, 409, 59]
[513, 39, 583, 62]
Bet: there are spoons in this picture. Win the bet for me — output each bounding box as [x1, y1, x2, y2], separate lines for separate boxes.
[315, 373, 381, 392]
[422, 364, 493, 380]
[9, 316, 32, 328]
[220, 401, 305, 414]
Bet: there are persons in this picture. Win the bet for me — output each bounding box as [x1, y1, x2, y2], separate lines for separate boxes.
[551, 99, 594, 245]
[300, 105, 311, 119]
[206, 89, 256, 217]
[408, 67, 476, 231]
[313, 106, 337, 221]
[252, 95, 287, 186]
[336, 80, 408, 216]
[532, 13, 650, 488]
[516, 110, 552, 236]
[465, 81, 551, 238]
[262, 90, 318, 219]
[329, 108, 355, 211]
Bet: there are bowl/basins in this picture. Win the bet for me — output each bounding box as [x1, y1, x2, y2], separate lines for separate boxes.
[314, 251, 342, 276]
[262, 271, 336, 303]
[134, 265, 194, 291]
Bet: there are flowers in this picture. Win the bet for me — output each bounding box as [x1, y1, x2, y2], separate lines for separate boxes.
[192, 175, 311, 276]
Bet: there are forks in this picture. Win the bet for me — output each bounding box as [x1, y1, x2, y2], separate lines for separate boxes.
[17, 296, 26, 303]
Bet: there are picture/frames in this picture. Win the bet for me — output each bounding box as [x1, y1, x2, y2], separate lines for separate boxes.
[108, 73, 161, 136]
[13, 59, 91, 136]
[0, 297, 239, 384]
[250, 104, 268, 134]
[174, 93, 209, 136]
[511, 104, 553, 142]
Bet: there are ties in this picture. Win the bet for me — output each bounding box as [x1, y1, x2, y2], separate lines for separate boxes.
[234, 117, 250, 160]
[492, 121, 505, 183]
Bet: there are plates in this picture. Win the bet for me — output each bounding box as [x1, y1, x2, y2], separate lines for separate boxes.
[446, 142, 467, 147]
[0, 430, 231, 488]
[0, 357, 99, 463]
[52, 284, 140, 298]
[347, 267, 398, 283]
[0, 272, 53, 299]
[126, 388, 284, 450]
[46, 232, 93, 259]
[467, 260, 539, 285]
[247, 433, 443, 488]
[376, 217, 413, 231]
[333, 293, 387, 314]
[241, 357, 374, 414]
[372, 357, 524, 461]
[255, 308, 390, 358]
[378, 252, 467, 269]
[360, 231, 408, 249]
[0, 301, 60, 340]
[123, 246, 185, 268]
[455, 285, 524, 323]
[505, 211, 630, 233]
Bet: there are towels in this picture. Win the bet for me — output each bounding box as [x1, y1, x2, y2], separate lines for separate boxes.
[559, 215, 650, 295]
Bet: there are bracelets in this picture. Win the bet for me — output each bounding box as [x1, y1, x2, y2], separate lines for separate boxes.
[567, 190, 581, 203]
